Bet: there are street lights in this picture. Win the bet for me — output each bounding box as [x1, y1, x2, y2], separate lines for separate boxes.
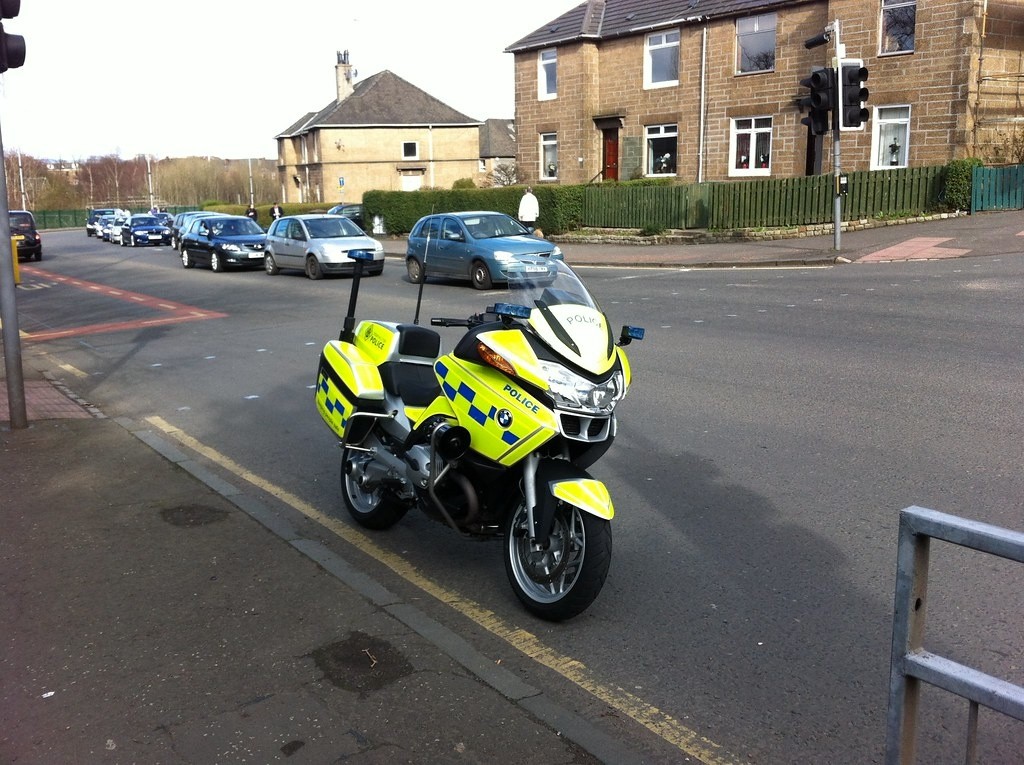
[14, 145, 26, 211]
[144, 154, 154, 210]
[810, 67, 834, 112]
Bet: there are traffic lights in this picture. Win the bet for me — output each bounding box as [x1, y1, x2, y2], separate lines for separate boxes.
[842, 62, 869, 127]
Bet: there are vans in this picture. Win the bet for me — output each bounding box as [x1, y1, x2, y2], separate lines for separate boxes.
[264, 213, 385, 280]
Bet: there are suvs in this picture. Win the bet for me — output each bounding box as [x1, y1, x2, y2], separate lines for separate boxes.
[8, 210, 42, 261]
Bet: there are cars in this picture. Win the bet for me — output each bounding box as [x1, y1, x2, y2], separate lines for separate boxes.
[85, 208, 174, 244]
[171, 211, 268, 272]
[327, 204, 367, 231]
[406, 210, 564, 290]
[119, 214, 171, 247]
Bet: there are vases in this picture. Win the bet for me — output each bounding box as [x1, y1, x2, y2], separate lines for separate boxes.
[760, 164, 769, 168]
[548, 171, 554, 178]
[742, 163, 748, 169]
[889, 152, 900, 165]
[660, 162, 667, 173]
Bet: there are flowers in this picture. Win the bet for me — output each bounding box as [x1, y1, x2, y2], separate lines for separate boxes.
[760, 155, 769, 163]
[740, 155, 750, 163]
[890, 139, 899, 153]
[548, 163, 556, 170]
[656, 151, 671, 164]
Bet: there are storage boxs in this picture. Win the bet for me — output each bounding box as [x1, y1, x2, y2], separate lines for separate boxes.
[315, 339, 385, 444]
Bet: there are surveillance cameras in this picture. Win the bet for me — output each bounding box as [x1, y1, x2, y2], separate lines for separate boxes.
[804, 33, 831, 49]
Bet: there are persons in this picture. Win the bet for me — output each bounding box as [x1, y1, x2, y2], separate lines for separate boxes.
[123, 208, 131, 217]
[518, 187, 539, 227]
[244, 204, 257, 222]
[270, 202, 283, 220]
[533, 225, 544, 238]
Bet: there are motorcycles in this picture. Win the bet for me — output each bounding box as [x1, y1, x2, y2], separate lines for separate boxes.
[314, 250, 645, 622]
